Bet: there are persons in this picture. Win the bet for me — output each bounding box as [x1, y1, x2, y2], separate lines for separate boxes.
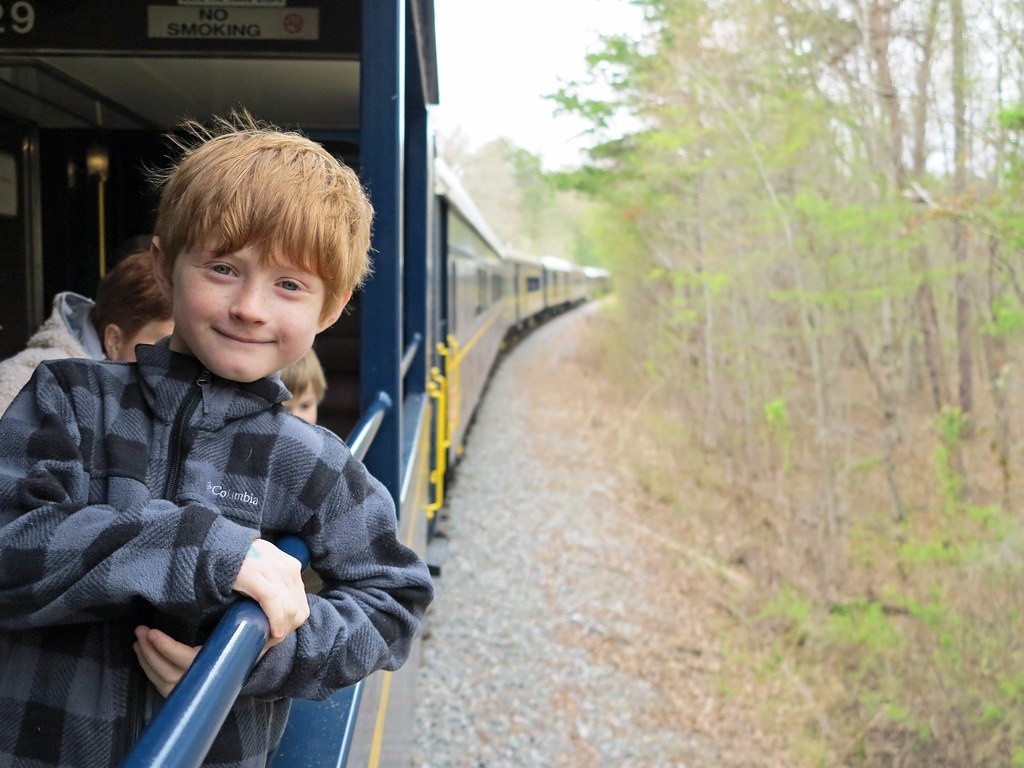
[0, 110, 436, 768]
[280, 347, 327, 424]
[0, 251, 175, 418]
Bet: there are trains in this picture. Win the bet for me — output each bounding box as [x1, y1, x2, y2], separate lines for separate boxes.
[0, 1, 609, 768]
